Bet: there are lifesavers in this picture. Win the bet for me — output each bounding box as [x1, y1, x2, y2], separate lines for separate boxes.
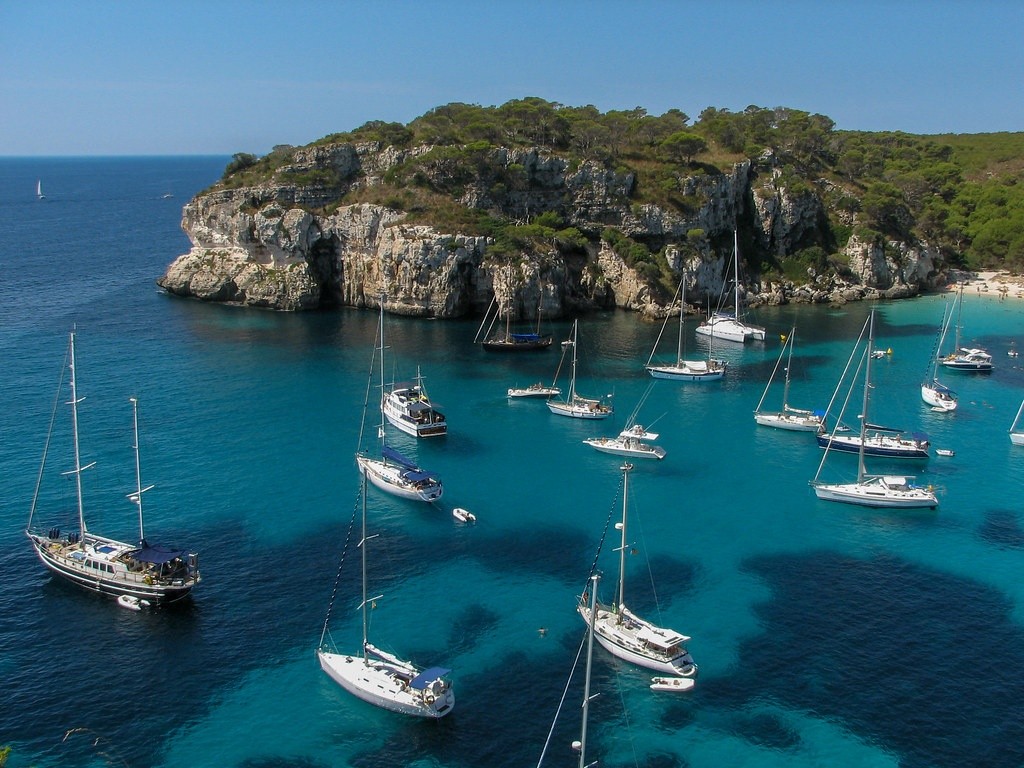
[144, 576, 152, 584]
[427, 695, 434, 704]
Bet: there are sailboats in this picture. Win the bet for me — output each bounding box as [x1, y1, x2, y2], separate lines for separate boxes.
[694, 229, 766, 344]
[354, 292, 444, 501]
[474, 256, 555, 351]
[317, 465, 457, 718]
[1006, 398, 1024, 447]
[922, 299, 959, 413]
[26, 316, 203, 616]
[753, 327, 827, 432]
[545, 316, 617, 420]
[574, 459, 701, 678]
[932, 281, 993, 371]
[816, 310, 932, 460]
[807, 350, 939, 509]
[642, 267, 731, 381]
[36, 179, 46, 199]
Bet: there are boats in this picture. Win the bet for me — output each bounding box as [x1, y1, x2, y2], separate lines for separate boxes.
[583, 379, 671, 460]
[452, 506, 477, 524]
[380, 364, 448, 439]
[649, 677, 696, 691]
[934, 449, 955, 457]
[508, 382, 560, 399]
